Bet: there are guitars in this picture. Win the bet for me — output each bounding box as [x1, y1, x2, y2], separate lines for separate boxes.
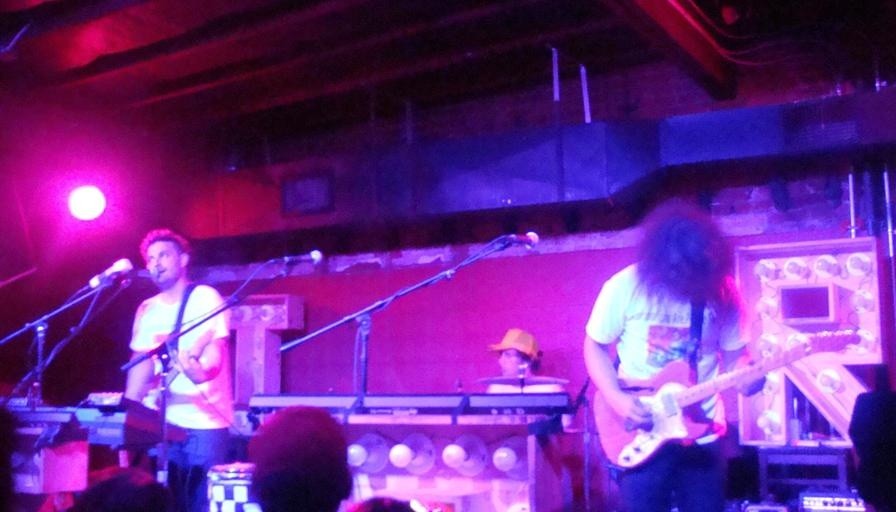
[594, 328, 865, 468]
[142, 329, 215, 410]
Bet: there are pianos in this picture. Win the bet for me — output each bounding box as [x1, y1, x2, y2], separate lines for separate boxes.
[1, 395, 189, 458]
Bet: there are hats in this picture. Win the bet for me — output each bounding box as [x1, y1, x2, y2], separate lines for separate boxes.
[486, 328, 540, 364]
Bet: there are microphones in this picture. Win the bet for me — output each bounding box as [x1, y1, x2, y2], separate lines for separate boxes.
[284, 250, 324, 265]
[500, 231, 540, 252]
[88, 258, 133, 290]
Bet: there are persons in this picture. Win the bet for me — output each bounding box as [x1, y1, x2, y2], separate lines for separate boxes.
[487, 327, 563, 394]
[246, 405, 351, 511]
[118, 227, 235, 511]
[850, 386, 896, 512]
[63, 465, 179, 511]
[582, 198, 767, 510]
[1, 407, 18, 511]
